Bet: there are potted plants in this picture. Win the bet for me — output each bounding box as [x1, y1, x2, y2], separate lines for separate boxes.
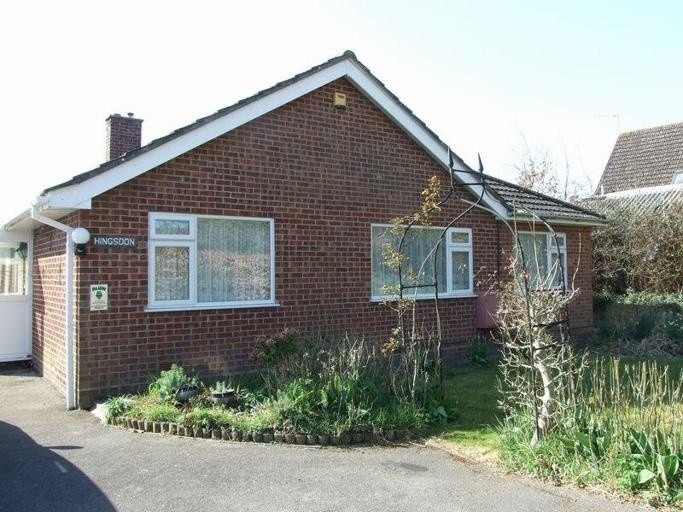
[212, 381, 235, 404]
[113, 413, 407, 445]
[155, 363, 197, 401]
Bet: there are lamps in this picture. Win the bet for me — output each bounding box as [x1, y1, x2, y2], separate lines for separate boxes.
[15, 242, 28, 258]
[70, 227, 90, 255]
